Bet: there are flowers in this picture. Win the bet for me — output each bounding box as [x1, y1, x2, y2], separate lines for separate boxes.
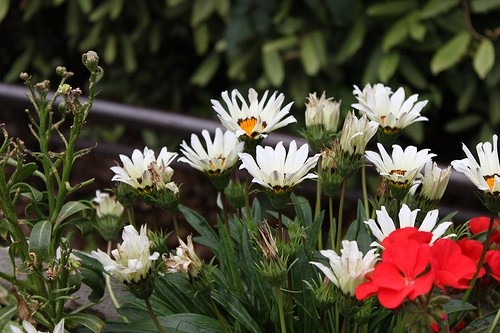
[1, 52, 499, 333]
